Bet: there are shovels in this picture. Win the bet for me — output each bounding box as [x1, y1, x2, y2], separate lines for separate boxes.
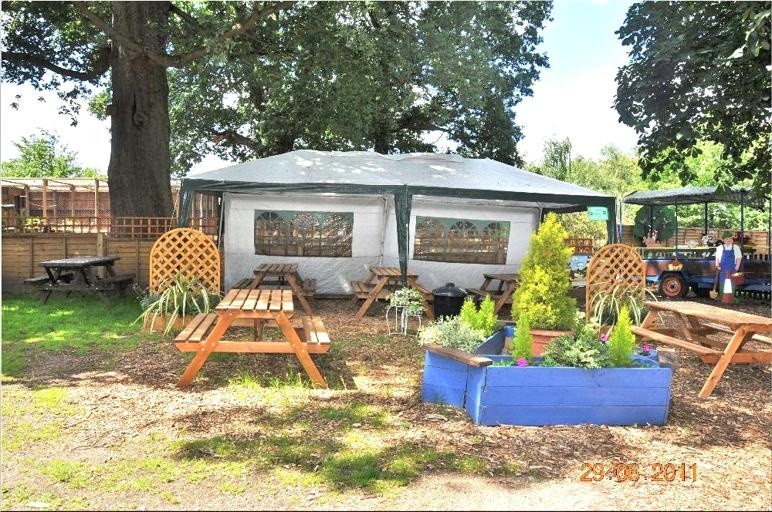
[709, 266, 720, 299]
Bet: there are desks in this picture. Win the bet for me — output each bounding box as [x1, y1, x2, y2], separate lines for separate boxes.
[176, 289, 327, 388]
[247, 263, 313, 315]
[630, 301, 772, 400]
[24, 273, 72, 299]
[480, 274, 521, 315]
[352, 268, 435, 320]
[37, 256, 121, 297]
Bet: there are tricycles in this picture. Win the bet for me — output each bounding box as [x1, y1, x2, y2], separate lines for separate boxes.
[602, 185, 752, 300]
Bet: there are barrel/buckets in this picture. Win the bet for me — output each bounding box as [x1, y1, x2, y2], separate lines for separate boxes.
[731, 270, 744, 285]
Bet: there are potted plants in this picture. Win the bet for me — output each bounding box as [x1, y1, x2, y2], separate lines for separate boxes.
[511, 211, 576, 354]
[386, 287, 426, 306]
[418, 293, 506, 408]
[403, 301, 427, 320]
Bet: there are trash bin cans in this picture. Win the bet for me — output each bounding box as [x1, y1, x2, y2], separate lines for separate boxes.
[432, 283, 467, 323]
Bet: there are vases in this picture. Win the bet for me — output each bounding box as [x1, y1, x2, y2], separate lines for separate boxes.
[464, 356, 672, 425]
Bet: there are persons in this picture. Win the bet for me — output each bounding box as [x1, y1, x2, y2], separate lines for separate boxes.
[715, 231, 742, 304]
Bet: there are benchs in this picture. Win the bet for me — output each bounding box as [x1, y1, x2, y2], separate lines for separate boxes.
[94, 273, 136, 298]
[417, 285, 434, 300]
[701, 323, 772, 345]
[302, 278, 317, 296]
[172, 313, 219, 352]
[293, 315, 331, 353]
[351, 280, 369, 298]
[466, 288, 499, 302]
[37, 285, 115, 306]
[625, 325, 725, 363]
[231, 278, 254, 289]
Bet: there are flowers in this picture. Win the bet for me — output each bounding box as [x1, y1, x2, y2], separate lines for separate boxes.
[600, 334, 607, 341]
[518, 358, 528, 367]
[643, 344, 650, 352]
